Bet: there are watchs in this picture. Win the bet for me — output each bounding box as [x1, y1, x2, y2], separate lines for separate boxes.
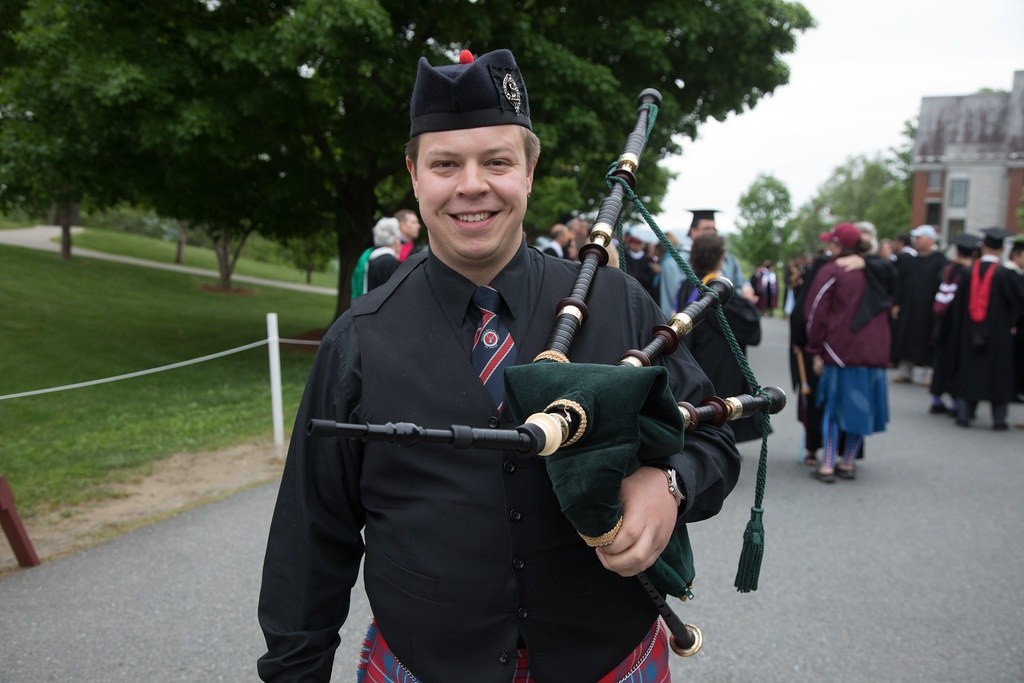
[641, 463, 687, 507]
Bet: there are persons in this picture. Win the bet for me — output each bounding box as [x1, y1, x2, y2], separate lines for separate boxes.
[255, 48, 742, 683]
[353, 208, 1024, 483]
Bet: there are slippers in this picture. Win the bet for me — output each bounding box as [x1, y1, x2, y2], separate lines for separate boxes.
[835, 463, 855, 479]
[810, 469, 834, 483]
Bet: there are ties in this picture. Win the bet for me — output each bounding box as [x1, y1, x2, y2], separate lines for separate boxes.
[469, 284, 517, 416]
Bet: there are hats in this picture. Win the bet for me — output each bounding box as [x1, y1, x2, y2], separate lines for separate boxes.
[689, 209, 719, 220]
[980, 226, 1012, 248]
[909, 225, 937, 239]
[1010, 238, 1024, 251]
[411, 49, 533, 138]
[627, 236, 642, 243]
[559, 209, 579, 224]
[818, 223, 860, 249]
[950, 232, 982, 258]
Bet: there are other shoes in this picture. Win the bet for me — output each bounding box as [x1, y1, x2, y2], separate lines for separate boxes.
[947, 409, 974, 418]
[804, 454, 818, 466]
[994, 421, 1010, 431]
[918, 378, 931, 385]
[929, 402, 947, 414]
[892, 376, 913, 384]
[957, 416, 969, 428]
